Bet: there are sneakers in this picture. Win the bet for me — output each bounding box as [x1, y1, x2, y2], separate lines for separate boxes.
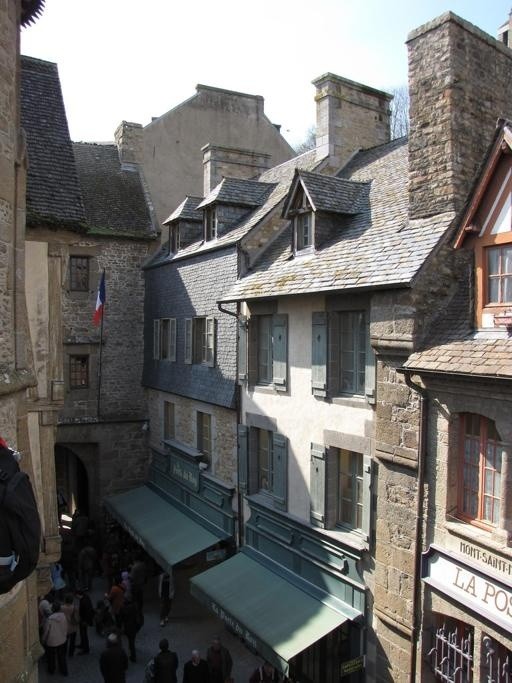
[74, 644, 90, 655]
[160, 616, 169, 627]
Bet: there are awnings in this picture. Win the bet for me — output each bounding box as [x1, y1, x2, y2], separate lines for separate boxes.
[106, 485, 235, 580]
[190, 551, 348, 678]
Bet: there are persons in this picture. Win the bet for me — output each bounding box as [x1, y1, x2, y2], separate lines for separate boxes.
[183, 636, 279, 682]
[41, 510, 179, 682]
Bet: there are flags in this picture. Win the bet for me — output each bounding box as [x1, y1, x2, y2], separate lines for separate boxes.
[94, 272, 106, 326]
